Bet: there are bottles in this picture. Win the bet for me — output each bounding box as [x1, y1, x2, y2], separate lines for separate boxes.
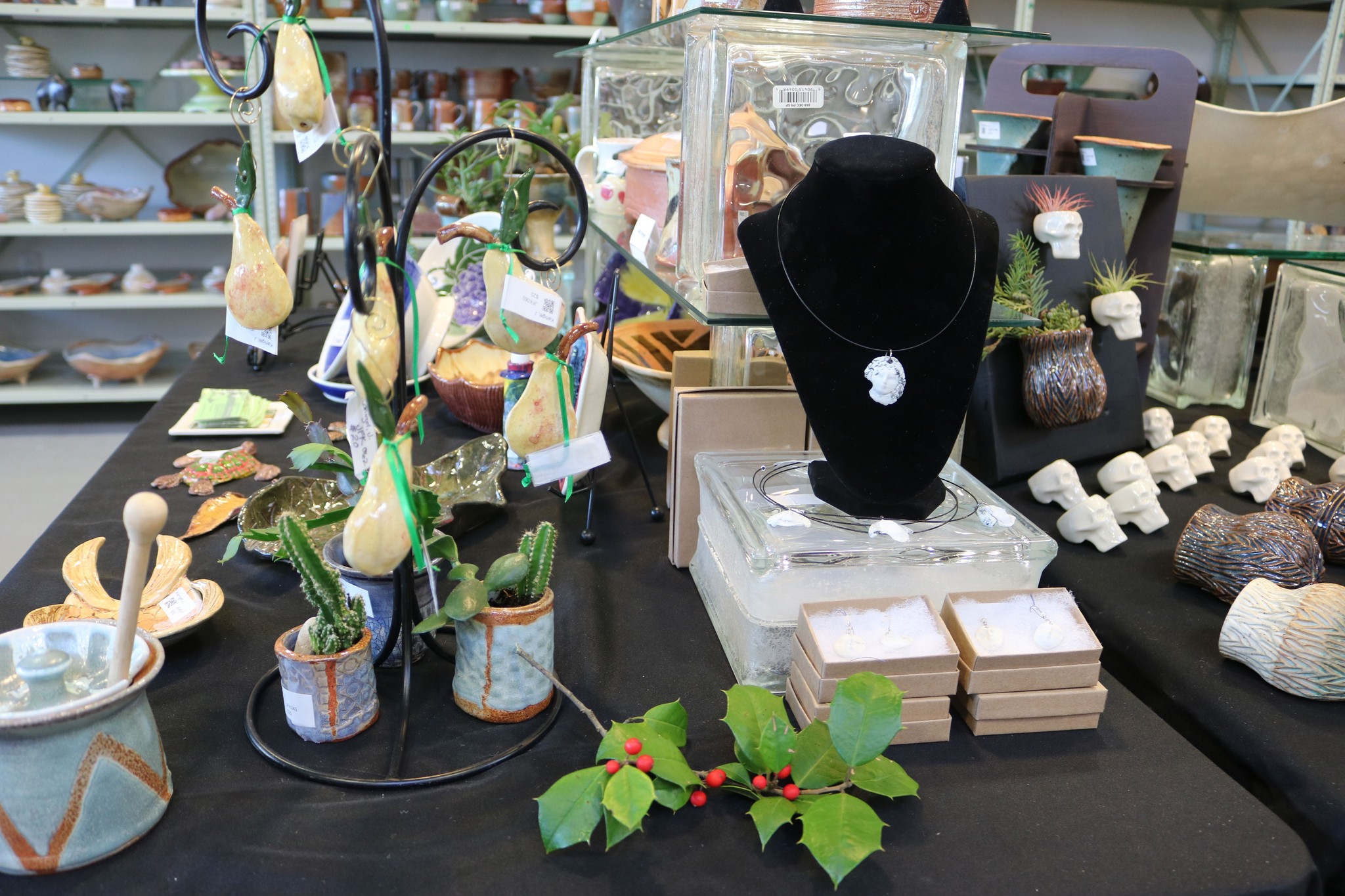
[499, 353, 537, 469]
[0, 169, 35, 221]
[24, 183, 63, 222]
[57, 172, 94, 221]
[5, 36, 52, 79]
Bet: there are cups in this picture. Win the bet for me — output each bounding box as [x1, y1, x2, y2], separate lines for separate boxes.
[655, 157, 680, 269]
[334, 95, 581, 134]
[574, 138, 642, 218]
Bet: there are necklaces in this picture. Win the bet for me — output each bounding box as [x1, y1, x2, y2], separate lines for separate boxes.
[776, 184, 976, 406]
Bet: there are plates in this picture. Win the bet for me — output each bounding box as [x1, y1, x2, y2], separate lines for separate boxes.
[307, 364, 356, 404]
[168, 401, 294, 434]
[558, 306, 609, 496]
[164, 137, 243, 214]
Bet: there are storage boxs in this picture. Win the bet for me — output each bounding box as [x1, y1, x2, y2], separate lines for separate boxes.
[786, 587, 1109, 745]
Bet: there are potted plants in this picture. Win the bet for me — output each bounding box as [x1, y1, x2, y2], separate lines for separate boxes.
[1023, 179, 1094, 259]
[481, 91, 584, 222]
[413, 521, 560, 721]
[1081, 247, 1169, 340]
[977, 230, 1106, 424]
[215, 361, 444, 667]
[273, 512, 380, 743]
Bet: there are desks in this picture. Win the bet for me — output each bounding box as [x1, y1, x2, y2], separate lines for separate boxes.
[968, 398, 1345, 896]
[0, 305, 1319, 896]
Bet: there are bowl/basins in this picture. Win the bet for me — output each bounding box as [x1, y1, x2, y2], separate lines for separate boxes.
[417, 211, 502, 346]
[120, 263, 156, 293]
[320, 51, 583, 99]
[0, 618, 174, 876]
[63, 335, 170, 385]
[426, 338, 510, 434]
[0, 345, 50, 385]
[202, 266, 228, 291]
[316, 246, 456, 381]
[598, 320, 711, 451]
[77, 186, 153, 223]
[40, 268, 75, 294]
[63, 272, 118, 295]
[0, 276, 40, 295]
[271, 0, 610, 28]
[152, 273, 196, 293]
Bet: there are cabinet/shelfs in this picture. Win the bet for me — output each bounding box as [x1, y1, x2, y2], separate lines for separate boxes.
[0, 0, 654, 403]
[555, 8, 1054, 384]
